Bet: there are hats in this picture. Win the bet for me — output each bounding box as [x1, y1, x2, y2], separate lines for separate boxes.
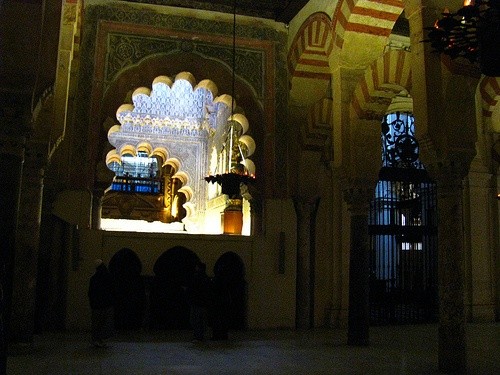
[94, 258, 103, 267]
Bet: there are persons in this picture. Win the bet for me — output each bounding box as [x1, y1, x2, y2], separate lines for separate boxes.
[231, 145, 245, 174]
[189, 261, 214, 342]
[89, 261, 117, 347]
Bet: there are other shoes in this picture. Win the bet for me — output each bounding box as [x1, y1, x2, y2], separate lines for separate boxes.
[88, 341, 106, 348]
